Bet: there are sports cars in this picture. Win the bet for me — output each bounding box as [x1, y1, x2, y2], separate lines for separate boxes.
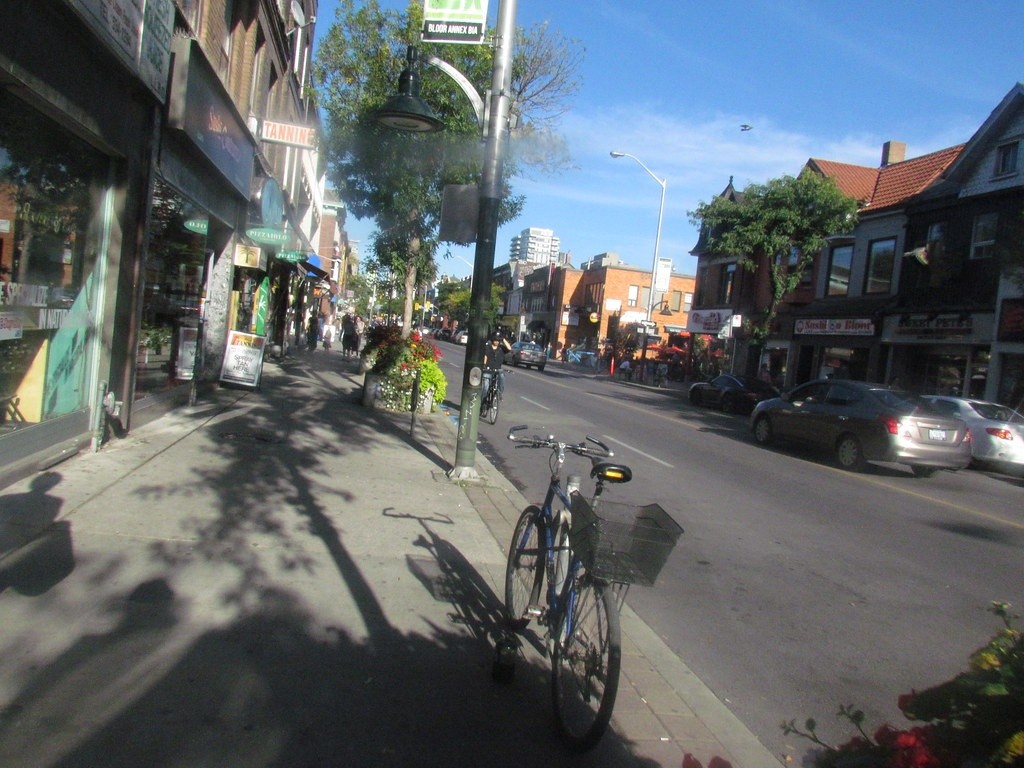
[688, 373, 779, 415]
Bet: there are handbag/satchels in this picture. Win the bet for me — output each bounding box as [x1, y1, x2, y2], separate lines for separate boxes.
[626, 366, 632, 374]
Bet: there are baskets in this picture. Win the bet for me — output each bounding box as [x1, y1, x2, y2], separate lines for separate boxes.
[570, 490, 684, 587]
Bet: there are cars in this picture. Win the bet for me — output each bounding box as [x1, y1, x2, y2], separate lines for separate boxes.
[748, 378, 974, 477]
[417, 326, 468, 345]
[505, 341, 546, 372]
[892, 394, 1024, 471]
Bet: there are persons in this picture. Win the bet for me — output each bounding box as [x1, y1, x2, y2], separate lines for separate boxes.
[531, 337, 723, 389]
[756, 365, 775, 387]
[889, 376, 1009, 408]
[508, 334, 516, 345]
[480, 329, 511, 416]
[338, 313, 386, 364]
[294, 302, 333, 352]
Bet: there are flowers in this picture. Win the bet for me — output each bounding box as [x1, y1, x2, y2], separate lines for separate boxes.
[374, 333, 448, 413]
[363, 323, 400, 354]
[140, 325, 173, 354]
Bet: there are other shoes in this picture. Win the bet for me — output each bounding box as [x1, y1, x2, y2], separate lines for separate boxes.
[499, 392, 504, 401]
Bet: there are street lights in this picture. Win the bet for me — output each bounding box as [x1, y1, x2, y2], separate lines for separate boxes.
[609, 151, 674, 380]
[456, 254, 474, 297]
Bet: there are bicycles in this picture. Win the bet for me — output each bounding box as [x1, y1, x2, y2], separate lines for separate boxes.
[481, 366, 513, 425]
[504, 425, 683, 754]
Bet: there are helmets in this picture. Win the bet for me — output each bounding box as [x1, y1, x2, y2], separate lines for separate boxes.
[491, 330, 503, 341]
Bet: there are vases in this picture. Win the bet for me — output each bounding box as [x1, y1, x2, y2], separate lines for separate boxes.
[137, 343, 171, 369]
